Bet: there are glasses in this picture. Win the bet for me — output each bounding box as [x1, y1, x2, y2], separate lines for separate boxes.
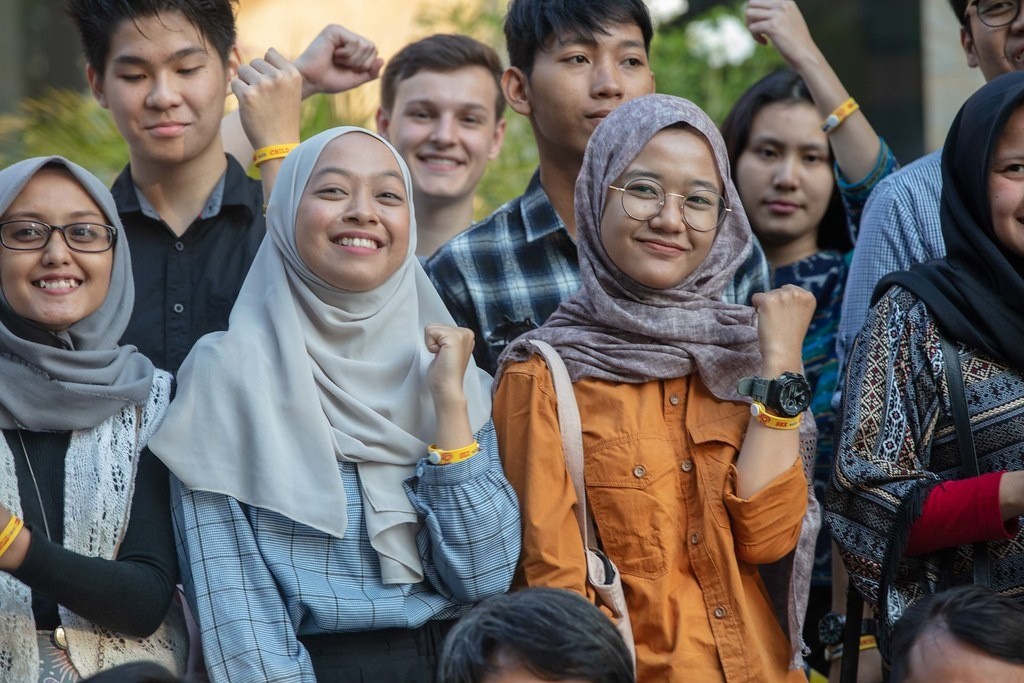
[609, 179, 732, 233]
[964, 0, 1021, 28]
[0, 220, 118, 253]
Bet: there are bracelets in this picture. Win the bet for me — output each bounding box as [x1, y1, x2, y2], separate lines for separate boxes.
[0, 513, 25, 556]
[826, 634, 876, 664]
[749, 401, 805, 429]
[817, 96, 859, 136]
[427, 439, 479, 465]
[252, 143, 299, 168]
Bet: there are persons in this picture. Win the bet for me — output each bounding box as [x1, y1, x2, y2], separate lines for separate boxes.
[0, 0, 1024, 683]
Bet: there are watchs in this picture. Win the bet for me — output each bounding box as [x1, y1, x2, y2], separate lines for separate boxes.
[817, 610, 876, 648]
[736, 371, 815, 419]
